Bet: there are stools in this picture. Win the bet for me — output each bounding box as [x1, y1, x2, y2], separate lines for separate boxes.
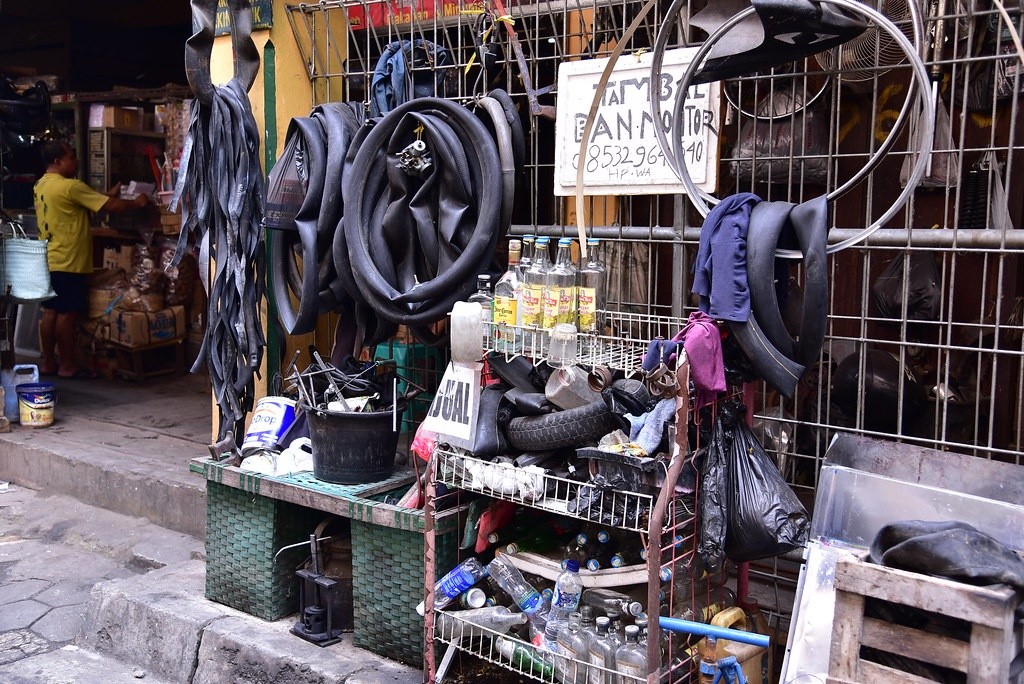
[371, 342, 442, 434]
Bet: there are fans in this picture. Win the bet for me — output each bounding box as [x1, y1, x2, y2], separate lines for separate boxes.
[814, 0, 914, 82]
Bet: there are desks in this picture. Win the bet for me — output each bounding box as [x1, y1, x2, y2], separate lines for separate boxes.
[113, 336, 188, 384]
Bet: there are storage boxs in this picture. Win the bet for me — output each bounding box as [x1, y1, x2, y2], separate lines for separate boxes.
[105, 105, 140, 130]
[823, 550, 1024, 684]
[109, 304, 187, 348]
[88, 289, 115, 311]
[90, 152, 118, 174]
[91, 132, 121, 151]
[102, 243, 160, 271]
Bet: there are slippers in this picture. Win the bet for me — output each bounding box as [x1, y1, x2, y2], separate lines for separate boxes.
[58, 367, 95, 380]
[39, 363, 59, 376]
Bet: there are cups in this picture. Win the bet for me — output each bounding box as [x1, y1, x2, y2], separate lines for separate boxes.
[546, 323, 577, 368]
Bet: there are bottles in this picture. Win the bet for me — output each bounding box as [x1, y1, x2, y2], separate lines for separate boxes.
[469, 227, 606, 352]
[414, 519, 733, 684]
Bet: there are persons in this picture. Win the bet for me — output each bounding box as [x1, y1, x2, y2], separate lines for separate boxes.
[34, 140, 149, 381]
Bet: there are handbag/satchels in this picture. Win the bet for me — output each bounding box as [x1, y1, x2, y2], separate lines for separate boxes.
[0, 221, 58, 303]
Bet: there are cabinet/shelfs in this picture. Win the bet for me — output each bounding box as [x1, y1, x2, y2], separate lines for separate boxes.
[86, 126, 166, 226]
[416, 306, 756, 684]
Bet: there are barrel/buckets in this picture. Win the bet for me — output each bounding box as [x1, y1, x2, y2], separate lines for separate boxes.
[296, 359, 407, 482]
[0, 364, 39, 422]
[16, 384, 58, 429]
[241, 396, 297, 451]
[696, 596, 774, 684]
